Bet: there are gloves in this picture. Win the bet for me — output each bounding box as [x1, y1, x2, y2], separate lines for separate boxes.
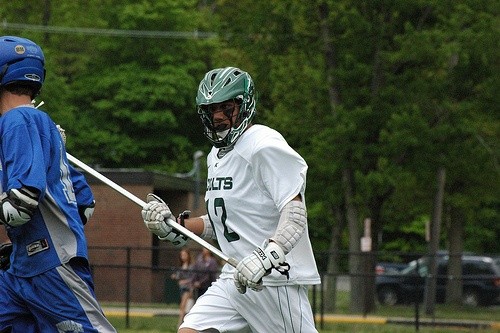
[141, 193, 191, 248]
[234, 242, 290, 294]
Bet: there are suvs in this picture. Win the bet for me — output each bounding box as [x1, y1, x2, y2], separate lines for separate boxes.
[374, 253, 500, 307]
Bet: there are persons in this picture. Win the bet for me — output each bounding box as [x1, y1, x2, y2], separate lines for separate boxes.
[0, 35, 118, 333]
[171, 246, 219, 328]
[140, 67, 322, 333]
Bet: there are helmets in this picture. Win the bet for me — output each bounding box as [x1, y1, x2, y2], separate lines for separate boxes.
[196, 66, 256, 148]
[0, 36, 46, 100]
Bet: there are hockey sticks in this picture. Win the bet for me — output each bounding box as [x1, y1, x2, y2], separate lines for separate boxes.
[55, 125, 262, 283]
